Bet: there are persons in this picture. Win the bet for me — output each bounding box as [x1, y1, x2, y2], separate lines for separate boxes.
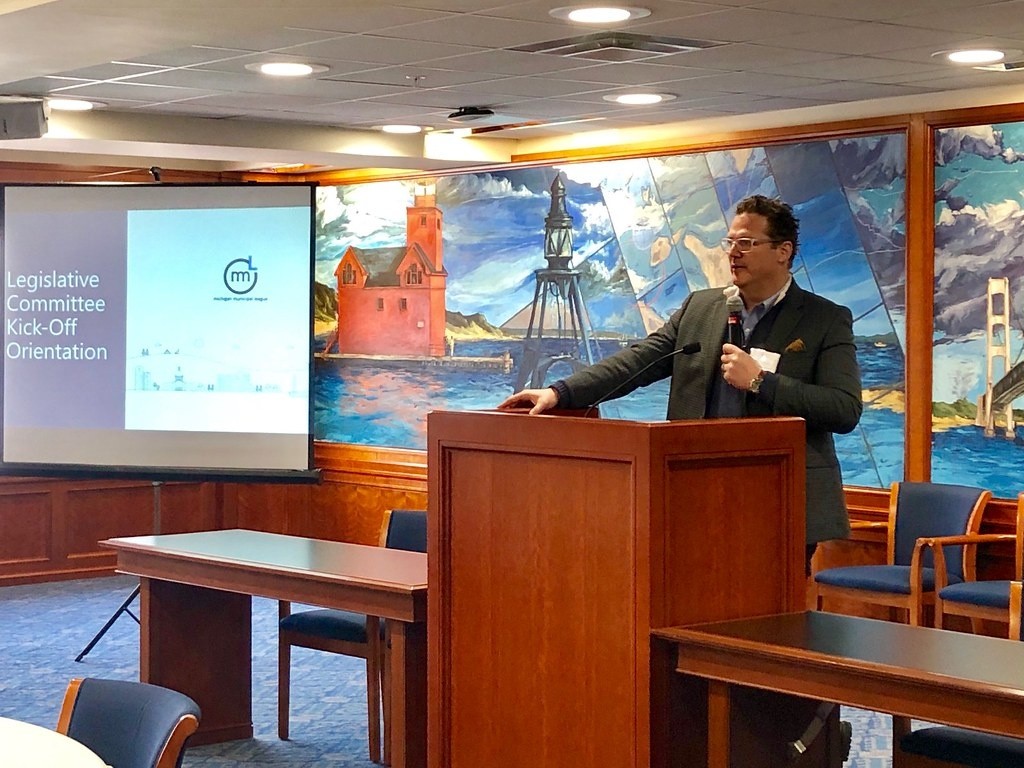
[496, 194, 864, 768]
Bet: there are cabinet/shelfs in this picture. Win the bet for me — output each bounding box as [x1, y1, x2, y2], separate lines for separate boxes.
[425, 403, 811, 766]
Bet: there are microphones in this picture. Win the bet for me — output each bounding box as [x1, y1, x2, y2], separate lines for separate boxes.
[584, 341, 702, 418]
[726, 295, 743, 351]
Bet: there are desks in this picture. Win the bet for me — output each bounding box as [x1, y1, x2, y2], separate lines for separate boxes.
[650, 610, 1024, 767]
[95, 526, 427, 768]
[0, 716, 112, 768]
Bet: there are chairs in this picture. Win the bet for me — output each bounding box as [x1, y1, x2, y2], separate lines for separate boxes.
[812, 482, 992, 637]
[276, 507, 427, 762]
[889, 716, 1024, 768]
[928, 492, 1024, 642]
[54, 677, 201, 768]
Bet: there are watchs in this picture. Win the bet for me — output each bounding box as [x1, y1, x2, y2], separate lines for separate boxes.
[748, 369, 765, 393]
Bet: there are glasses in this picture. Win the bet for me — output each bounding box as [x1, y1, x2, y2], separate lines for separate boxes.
[720, 238, 781, 252]
